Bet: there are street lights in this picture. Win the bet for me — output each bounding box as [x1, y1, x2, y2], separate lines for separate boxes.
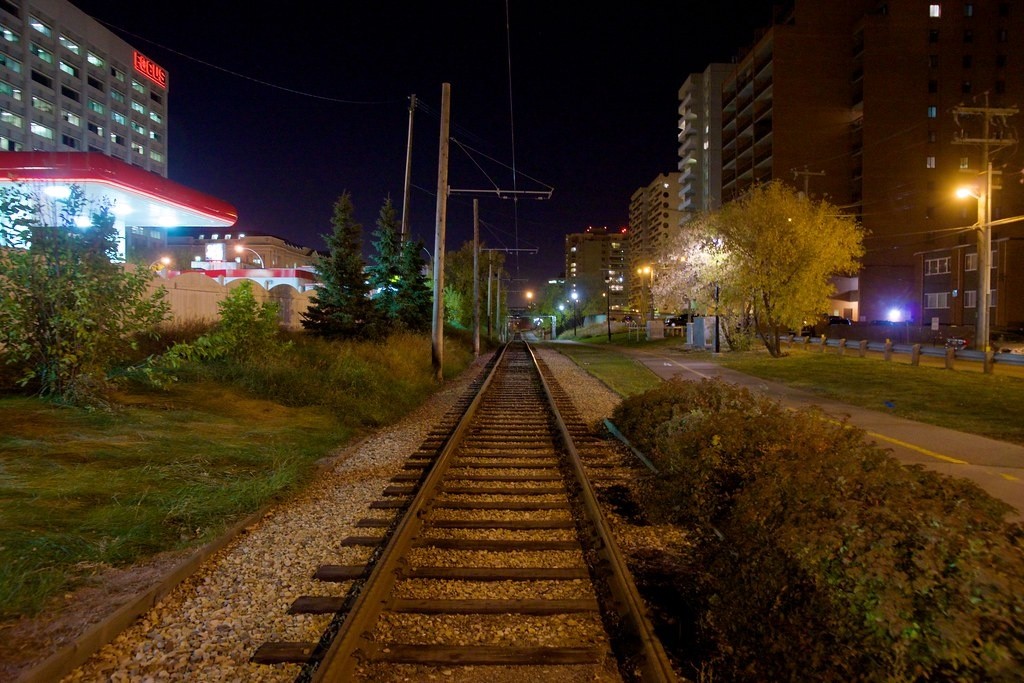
[645, 267, 654, 320]
[238, 247, 264, 269]
[713, 237, 723, 353]
[605, 277, 611, 341]
[406, 241, 433, 277]
[956, 187, 986, 350]
[573, 293, 577, 335]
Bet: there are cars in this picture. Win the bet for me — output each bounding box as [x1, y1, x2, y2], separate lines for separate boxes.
[944, 329, 1024, 354]
[870, 320, 904, 326]
[621, 315, 641, 324]
[735, 313, 754, 334]
[665, 313, 699, 327]
[788, 315, 854, 339]
